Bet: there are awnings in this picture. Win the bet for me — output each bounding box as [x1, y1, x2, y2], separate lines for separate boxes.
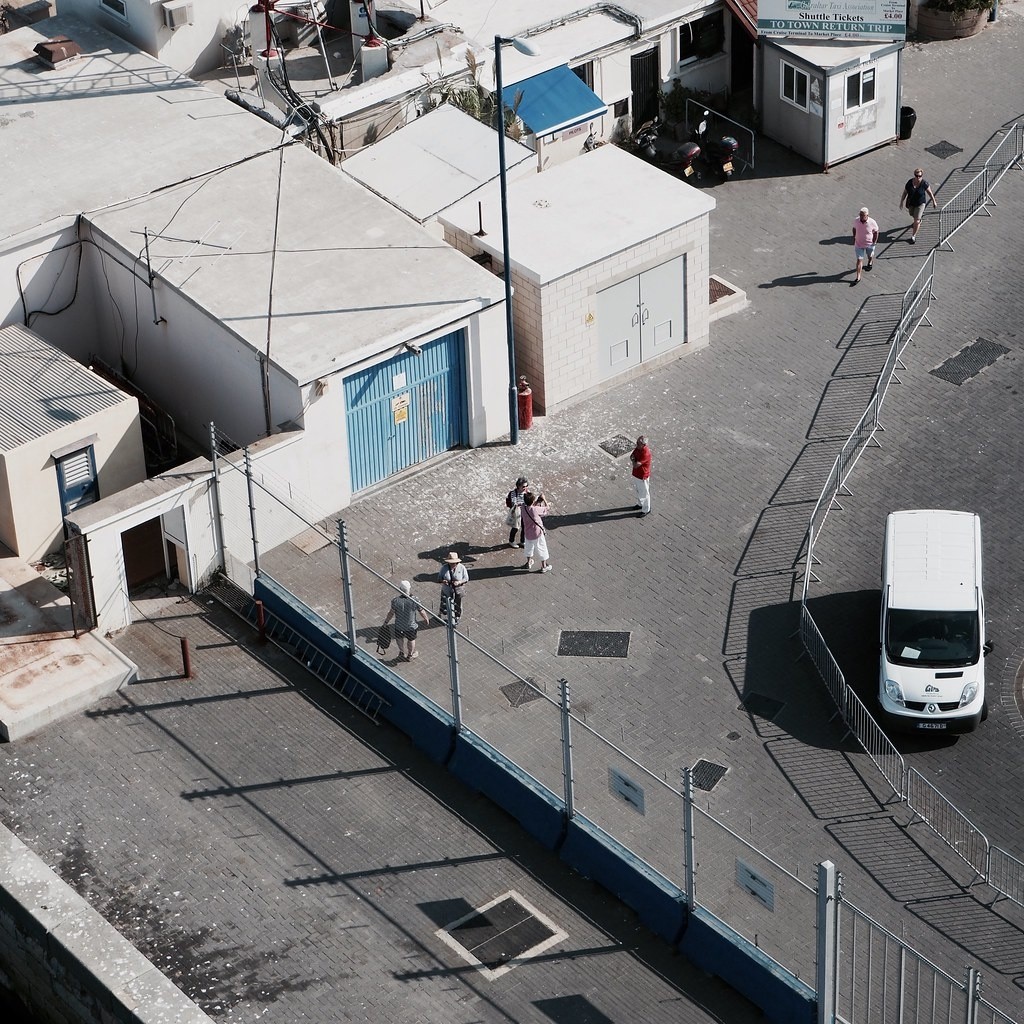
[499, 65, 608, 139]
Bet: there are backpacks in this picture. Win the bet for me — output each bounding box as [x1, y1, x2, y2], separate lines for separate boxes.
[376, 625, 391, 655]
[505, 489, 518, 509]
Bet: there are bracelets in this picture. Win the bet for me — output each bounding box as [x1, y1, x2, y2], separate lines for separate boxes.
[385, 621, 388, 623]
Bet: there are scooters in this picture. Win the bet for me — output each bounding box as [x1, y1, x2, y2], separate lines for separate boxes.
[583, 116, 701, 185]
[689, 110, 738, 182]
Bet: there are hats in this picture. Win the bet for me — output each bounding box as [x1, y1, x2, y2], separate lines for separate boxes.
[444, 552, 462, 564]
[400, 580, 411, 594]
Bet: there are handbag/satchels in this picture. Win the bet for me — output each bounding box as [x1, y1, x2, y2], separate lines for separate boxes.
[506, 503, 521, 529]
[542, 527, 547, 536]
[454, 586, 466, 597]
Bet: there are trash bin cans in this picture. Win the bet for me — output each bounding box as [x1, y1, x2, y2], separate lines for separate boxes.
[901, 106, 916, 140]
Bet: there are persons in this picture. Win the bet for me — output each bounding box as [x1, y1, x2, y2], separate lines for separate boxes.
[852, 207, 879, 286]
[630, 435, 651, 518]
[437, 552, 469, 625]
[900, 168, 937, 243]
[506, 477, 530, 548]
[521, 492, 552, 572]
[383, 580, 429, 662]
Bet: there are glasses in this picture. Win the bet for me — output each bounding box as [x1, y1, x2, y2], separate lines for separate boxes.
[519, 485, 528, 488]
[914, 174, 923, 178]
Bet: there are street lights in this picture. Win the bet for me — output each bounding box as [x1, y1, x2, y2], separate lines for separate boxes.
[495, 35, 542, 446]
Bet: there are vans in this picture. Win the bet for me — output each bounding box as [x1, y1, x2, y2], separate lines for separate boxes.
[879, 509, 995, 735]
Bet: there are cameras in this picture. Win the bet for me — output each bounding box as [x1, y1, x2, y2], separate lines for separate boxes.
[537, 496, 543, 503]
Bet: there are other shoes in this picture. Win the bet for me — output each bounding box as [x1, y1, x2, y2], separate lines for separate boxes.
[636, 510, 651, 518]
[398, 651, 407, 661]
[408, 651, 418, 662]
[521, 542, 526, 546]
[509, 541, 519, 548]
[910, 237, 915, 245]
[455, 618, 460, 625]
[526, 559, 535, 568]
[634, 504, 642, 510]
[866, 261, 873, 271]
[541, 565, 552, 573]
[849, 279, 860, 287]
